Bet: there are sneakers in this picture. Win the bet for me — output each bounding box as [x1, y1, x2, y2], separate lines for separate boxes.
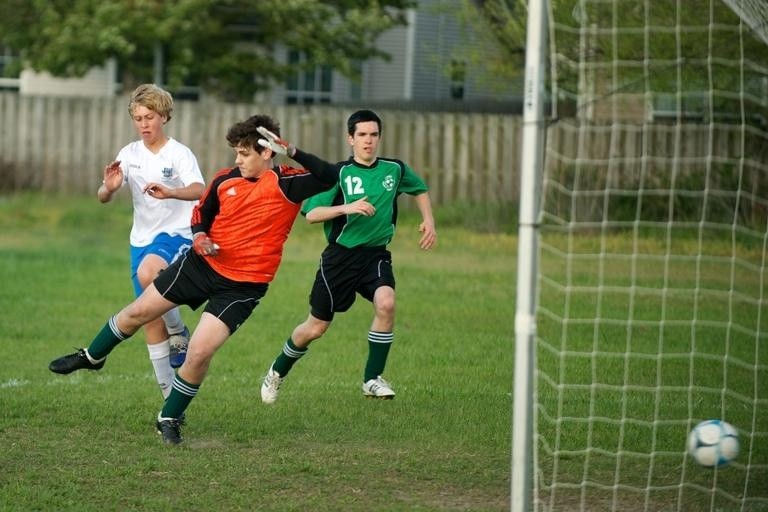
[361, 375, 396, 398]
[48, 348, 107, 375]
[153, 412, 186, 446]
[168, 325, 191, 369]
[260, 360, 284, 405]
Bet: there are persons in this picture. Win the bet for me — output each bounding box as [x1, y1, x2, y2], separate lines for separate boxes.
[99, 84, 206, 421]
[49, 111, 339, 447]
[260, 110, 437, 405]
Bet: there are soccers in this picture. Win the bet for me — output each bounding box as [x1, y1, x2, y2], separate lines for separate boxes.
[687, 419, 738, 467]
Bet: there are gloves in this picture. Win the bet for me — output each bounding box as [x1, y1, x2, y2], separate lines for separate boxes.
[192, 231, 218, 257]
[256, 125, 296, 159]
